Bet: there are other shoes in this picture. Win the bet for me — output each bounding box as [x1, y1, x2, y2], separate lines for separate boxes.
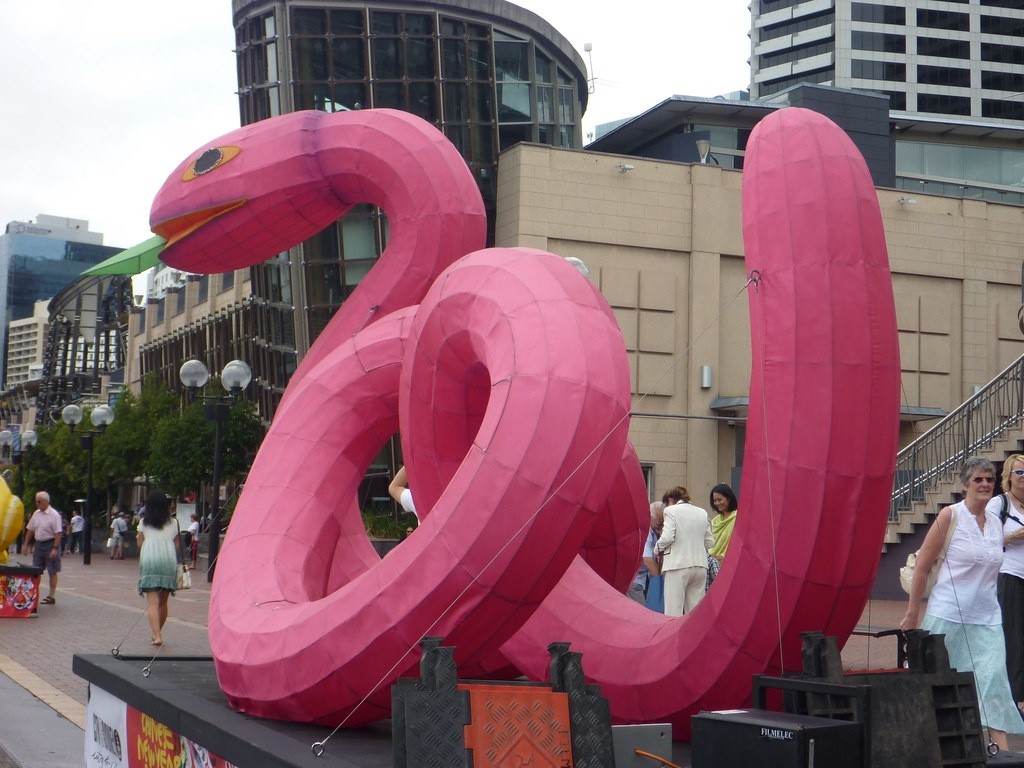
[110, 554, 125, 560]
[188, 565, 196, 569]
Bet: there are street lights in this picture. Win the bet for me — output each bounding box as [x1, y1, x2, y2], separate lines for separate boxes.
[179, 359, 253, 583]
[62, 404, 116, 565]
[0, 430, 38, 555]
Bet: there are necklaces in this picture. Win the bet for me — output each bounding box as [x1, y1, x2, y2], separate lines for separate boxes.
[1010, 491, 1024, 509]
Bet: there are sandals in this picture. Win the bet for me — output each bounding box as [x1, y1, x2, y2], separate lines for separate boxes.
[40, 596, 56, 604]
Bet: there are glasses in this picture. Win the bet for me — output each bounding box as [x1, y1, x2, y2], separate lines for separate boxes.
[36, 499, 45, 504]
[1011, 469, 1024, 477]
[653, 516, 663, 526]
[966, 477, 996, 484]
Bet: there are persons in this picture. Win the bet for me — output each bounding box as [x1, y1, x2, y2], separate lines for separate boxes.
[24, 489, 213, 571]
[21, 491, 62, 605]
[986, 454, 1024, 717]
[136, 490, 181, 645]
[388, 466, 420, 527]
[900, 456, 1024, 751]
[398, 526, 413, 545]
[641, 483, 738, 619]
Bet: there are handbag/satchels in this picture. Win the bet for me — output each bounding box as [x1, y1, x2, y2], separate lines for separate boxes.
[119, 531, 126, 536]
[900, 506, 959, 598]
[645, 575, 665, 613]
[107, 533, 113, 547]
[175, 564, 192, 589]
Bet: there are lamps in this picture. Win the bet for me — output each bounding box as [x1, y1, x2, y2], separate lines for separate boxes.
[618, 162, 634, 176]
[900, 197, 916, 205]
[700, 366, 712, 388]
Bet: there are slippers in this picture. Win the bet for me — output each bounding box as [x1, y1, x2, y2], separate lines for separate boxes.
[150, 634, 163, 645]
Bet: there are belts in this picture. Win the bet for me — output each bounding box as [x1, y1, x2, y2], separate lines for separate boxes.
[36, 538, 55, 544]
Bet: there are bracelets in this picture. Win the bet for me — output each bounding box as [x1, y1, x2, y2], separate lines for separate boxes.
[53, 545, 59, 548]
[1009, 532, 1014, 544]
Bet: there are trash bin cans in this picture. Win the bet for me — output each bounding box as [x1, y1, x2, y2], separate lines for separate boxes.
[175, 530, 190, 562]
[124, 530, 139, 557]
